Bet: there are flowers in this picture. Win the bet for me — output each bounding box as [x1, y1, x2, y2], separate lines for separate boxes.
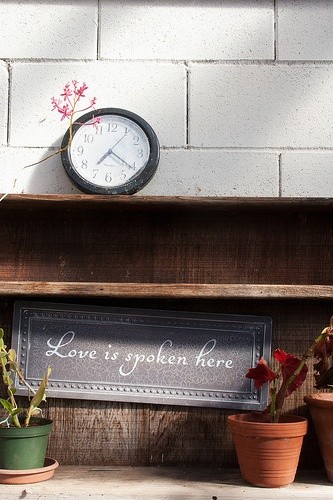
[0, 79, 101, 193]
[247, 316, 333, 420]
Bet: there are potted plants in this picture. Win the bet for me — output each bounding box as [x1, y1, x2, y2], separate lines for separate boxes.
[0, 325, 54, 469]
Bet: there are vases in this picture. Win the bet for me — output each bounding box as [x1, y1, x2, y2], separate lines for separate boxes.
[305, 390, 333, 483]
[228, 413, 306, 487]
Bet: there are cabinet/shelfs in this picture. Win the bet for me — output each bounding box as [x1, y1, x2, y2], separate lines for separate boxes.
[0, 191, 333, 305]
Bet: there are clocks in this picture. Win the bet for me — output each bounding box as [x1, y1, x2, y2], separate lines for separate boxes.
[61, 105, 162, 195]
